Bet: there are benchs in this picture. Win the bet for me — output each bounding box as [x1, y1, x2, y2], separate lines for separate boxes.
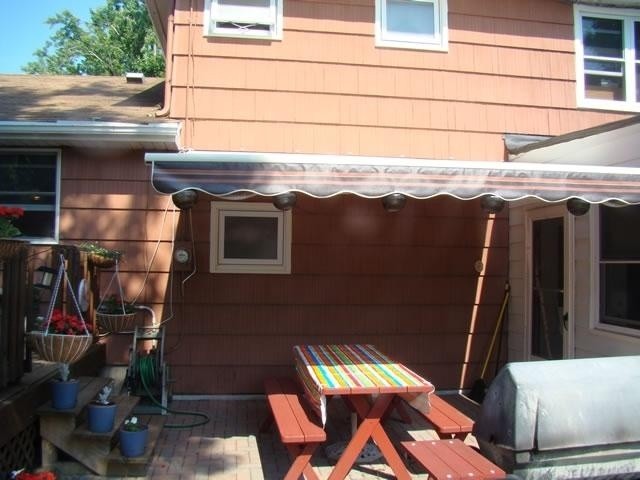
[259, 378, 508, 480]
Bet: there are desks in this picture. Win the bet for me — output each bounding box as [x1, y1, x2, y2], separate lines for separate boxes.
[292, 344, 435, 480]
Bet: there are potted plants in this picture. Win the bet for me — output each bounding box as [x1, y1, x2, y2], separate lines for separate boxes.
[86, 387, 116, 432]
[76, 242, 125, 268]
[47, 363, 80, 411]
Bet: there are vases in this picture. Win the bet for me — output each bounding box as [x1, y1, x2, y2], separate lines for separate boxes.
[119, 425, 149, 457]
[29, 333, 94, 362]
[96, 314, 137, 333]
[1, 239, 30, 263]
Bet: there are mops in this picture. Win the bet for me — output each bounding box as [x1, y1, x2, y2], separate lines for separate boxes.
[467, 291, 511, 401]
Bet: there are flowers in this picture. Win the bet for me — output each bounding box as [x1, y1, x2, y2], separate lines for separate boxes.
[124, 416, 139, 431]
[33, 309, 92, 336]
[1, 206, 24, 238]
[101, 293, 133, 313]
